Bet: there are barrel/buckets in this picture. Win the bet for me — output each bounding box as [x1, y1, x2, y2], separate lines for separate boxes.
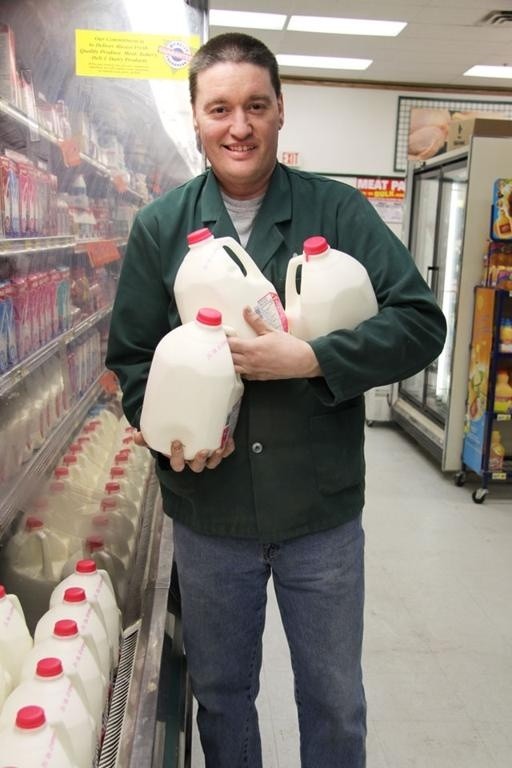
[47, 559, 122, 670]
[138, 307, 245, 461]
[92, 483, 136, 518]
[0, 582, 32, 680]
[16, 496, 69, 536]
[104, 452, 144, 485]
[58, 401, 143, 461]
[32, 586, 111, 683]
[41, 465, 87, 494]
[62, 536, 126, 610]
[1, 705, 74, 767]
[4, 657, 94, 767]
[32, 481, 73, 518]
[282, 235, 380, 344]
[3, 515, 69, 638]
[18, 618, 105, 725]
[78, 497, 134, 542]
[72, 515, 130, 561]
[48, 455, 94, 486]
[174, 229, 287, 341]
[95, 466, 140, 501]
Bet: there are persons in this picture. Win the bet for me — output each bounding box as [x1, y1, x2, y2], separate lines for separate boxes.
[105, 34, 447, 768]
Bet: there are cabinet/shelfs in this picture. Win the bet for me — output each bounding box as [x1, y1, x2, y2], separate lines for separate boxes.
[0, 2, 195, 767]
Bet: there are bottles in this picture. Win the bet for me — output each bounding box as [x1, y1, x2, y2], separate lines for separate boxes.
[499, 316, 512, 352]
[490, 429, 504, 470]
[71, 171, 87, 201]
[481, 245, 512, 291]
[495, 369, 509, 414]
